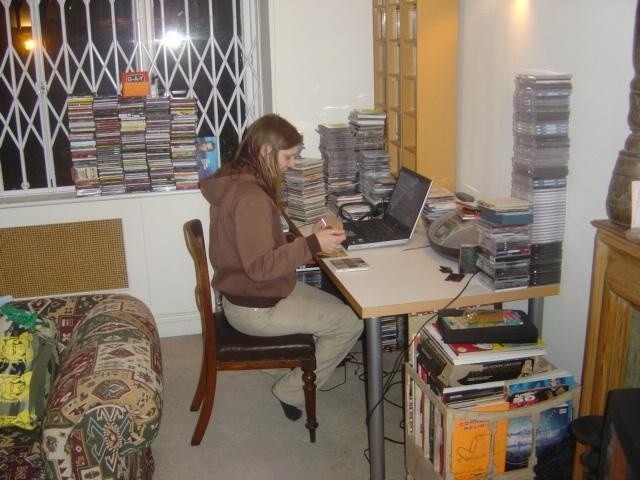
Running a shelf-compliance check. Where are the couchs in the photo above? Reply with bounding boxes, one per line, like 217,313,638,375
0,295,165,480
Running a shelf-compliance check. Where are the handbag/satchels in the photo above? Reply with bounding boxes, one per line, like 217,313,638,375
0,303,60,430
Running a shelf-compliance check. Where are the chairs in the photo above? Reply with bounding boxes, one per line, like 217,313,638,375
183,219,318,446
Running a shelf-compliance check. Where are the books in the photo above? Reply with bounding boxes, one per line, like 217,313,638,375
381,309,575,480
67,94,200,197
295,266,322,289
281,111,397,224
328,258,370,273
421,186,459,223
476,71,572,292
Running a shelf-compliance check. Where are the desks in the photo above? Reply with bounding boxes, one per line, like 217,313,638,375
282,206,560,480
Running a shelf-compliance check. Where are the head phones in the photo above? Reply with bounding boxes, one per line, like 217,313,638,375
339,202,388,222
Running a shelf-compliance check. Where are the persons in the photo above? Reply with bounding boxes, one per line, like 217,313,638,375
197,140,215,154
199,112,364,421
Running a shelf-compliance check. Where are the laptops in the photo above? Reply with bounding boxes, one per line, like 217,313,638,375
339,167,433,252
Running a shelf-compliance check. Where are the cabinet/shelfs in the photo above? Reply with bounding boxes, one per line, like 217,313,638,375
373,1,458,193
